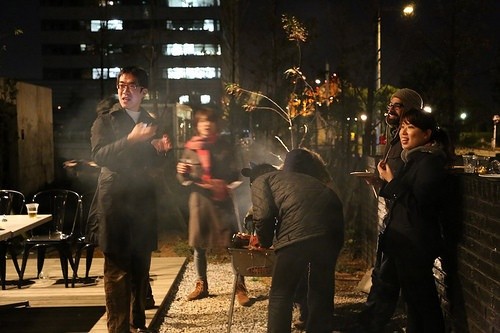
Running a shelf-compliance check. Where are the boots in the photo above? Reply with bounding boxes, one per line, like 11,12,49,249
235,285,250,306
188,278,208,299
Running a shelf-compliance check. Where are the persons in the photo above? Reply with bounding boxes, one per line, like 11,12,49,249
280,149,333,328
354,88,449,333
175,109,250,306
249,163,344,333
82,66,176,333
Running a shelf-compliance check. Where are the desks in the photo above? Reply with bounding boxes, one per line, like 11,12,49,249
0,215,52,286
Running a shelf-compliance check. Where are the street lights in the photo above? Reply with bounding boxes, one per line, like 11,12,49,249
377,2,415,143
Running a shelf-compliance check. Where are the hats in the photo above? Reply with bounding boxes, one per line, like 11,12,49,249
391,88,422,110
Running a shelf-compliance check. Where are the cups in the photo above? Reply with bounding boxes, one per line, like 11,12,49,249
462,154,476,173
26,204,39,219
475,156,490,174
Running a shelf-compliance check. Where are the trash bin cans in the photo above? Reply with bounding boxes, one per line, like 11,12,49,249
61,160,101,236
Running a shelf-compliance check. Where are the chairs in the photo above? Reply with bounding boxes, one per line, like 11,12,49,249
0,189,99,290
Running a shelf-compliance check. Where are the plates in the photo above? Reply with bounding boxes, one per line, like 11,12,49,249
478,173,500,178
350,171,374,176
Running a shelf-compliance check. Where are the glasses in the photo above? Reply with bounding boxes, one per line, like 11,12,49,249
116,84,144,90
386,103,404,110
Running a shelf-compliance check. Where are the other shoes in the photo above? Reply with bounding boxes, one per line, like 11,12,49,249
144,295,156,309
